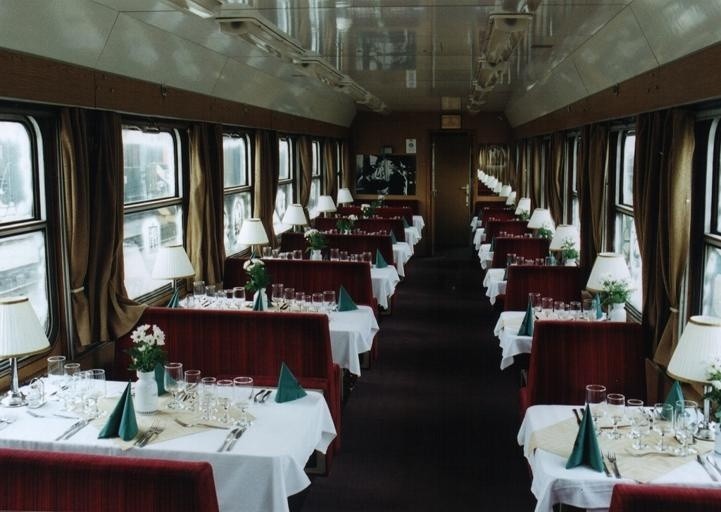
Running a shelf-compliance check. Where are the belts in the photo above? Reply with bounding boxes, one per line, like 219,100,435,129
525,291,598,322
199,376,219,421
25,354,107,427
232,377,254,427
625,398,645,441
605,394,625,440
651,402,674,455
670,399,699,456
181,369,201,413
270,282,337,320
188,279,247,313
214,379,235,423
627,410,650,452
162,362,184,409
584,384,605,439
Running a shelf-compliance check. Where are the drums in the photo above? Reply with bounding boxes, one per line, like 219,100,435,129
696,454,718,483
214,428,239,452
225,428,246,452
63,420,93,439
53,420,80,442
571,408,582,428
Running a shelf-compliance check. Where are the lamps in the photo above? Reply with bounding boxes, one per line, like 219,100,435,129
514,197,531,216
337,188,355,208
214,9,391,115
466,14,532,114
334,2,353,34
505,191,517,206
317,195,337,219
477,168,512,197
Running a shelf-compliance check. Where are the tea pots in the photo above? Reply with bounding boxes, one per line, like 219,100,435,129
477,142,518,198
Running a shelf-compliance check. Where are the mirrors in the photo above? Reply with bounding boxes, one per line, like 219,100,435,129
477,142,518,198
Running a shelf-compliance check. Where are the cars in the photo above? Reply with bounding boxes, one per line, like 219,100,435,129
334,214,402,221
496,230,556,268
326,227,388,237
482,207,514,212
260,246,373,267
347,204,411,209
488,216,520,222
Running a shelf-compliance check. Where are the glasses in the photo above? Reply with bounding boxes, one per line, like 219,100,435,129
214,9,391,115
337,188,355,208
514,197,531,216
466,14,532,114
477,168,512,197
334,2,353,34
505,191,517,206
317,195,337,219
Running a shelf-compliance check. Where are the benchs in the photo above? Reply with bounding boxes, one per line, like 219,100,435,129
0,198,420,512
474,200,721,512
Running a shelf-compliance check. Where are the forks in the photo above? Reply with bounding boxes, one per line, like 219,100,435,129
173,419,231,431
258,390,274,403
137,428,162,447
598,450,611,478
707,452,721,474
608,451,621,479
132,426,156,446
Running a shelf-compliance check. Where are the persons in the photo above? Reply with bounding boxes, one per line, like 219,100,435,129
386,160,404,195
